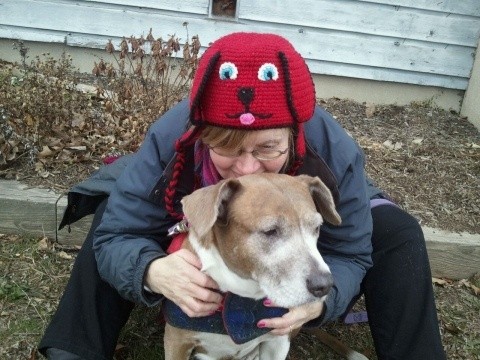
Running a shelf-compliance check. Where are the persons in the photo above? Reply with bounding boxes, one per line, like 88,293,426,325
30,32,451,359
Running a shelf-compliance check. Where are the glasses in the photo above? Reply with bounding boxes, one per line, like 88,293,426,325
207,144,289,161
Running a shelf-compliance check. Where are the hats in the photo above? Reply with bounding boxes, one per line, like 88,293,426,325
164,32,316,221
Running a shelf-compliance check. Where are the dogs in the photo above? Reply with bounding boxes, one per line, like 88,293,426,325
163,173,342,360
